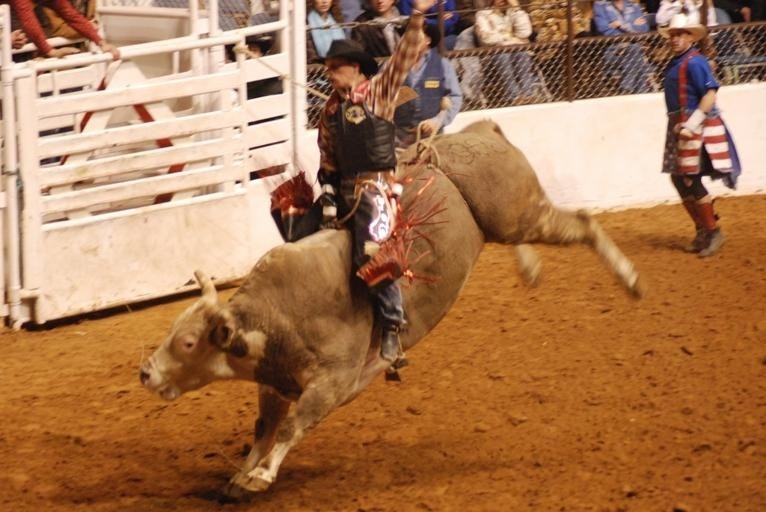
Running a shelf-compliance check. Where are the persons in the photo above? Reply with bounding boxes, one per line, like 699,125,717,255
3,1,120,76
594,1,653,95
225,2,461,124
656,0,765,85
451,0,547,107
395,15,464,147
272,1,435,368
659,11,744,262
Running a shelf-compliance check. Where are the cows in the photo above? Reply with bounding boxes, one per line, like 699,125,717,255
135,115,644,504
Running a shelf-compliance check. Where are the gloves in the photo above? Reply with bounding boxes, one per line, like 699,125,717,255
317,214,340,230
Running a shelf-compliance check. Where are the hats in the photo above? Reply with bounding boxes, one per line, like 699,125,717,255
395,20,439,48
658,15,705,41
307,39,378,75
226,35,273,61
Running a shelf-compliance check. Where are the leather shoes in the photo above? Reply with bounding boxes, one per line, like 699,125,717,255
380,323,399,362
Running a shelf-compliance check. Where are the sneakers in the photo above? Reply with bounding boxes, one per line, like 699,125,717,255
686,229,723,258
